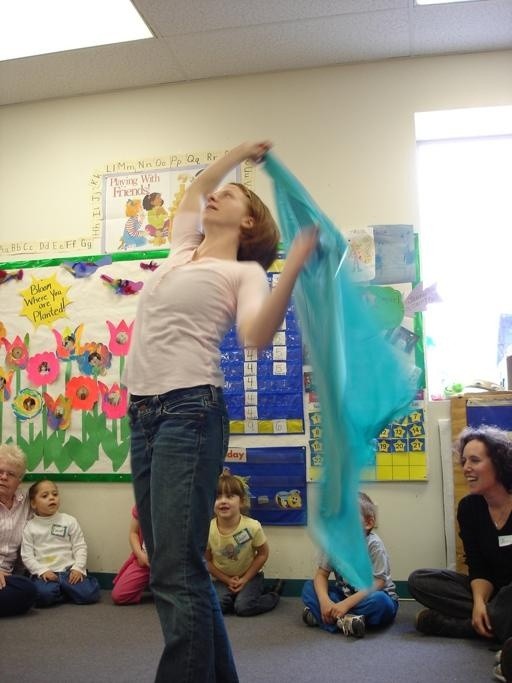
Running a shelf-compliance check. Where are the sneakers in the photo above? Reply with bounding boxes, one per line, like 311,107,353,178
302,607,317,626
335,612,365,637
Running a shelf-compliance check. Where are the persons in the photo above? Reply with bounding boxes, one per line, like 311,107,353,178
204,473,287,617
1,444,39,616
300,488,400,637
19,476,102,606
108,501,150,605
119,136,323,682
406,430,512,644
491,636,511,683
0,329,131,425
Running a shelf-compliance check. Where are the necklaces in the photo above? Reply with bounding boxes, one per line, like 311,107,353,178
486,502,509,528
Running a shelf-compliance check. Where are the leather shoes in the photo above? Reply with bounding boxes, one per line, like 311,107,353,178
416,609,456,639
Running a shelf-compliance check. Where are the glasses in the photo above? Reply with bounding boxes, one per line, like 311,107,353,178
0,469,19,478
